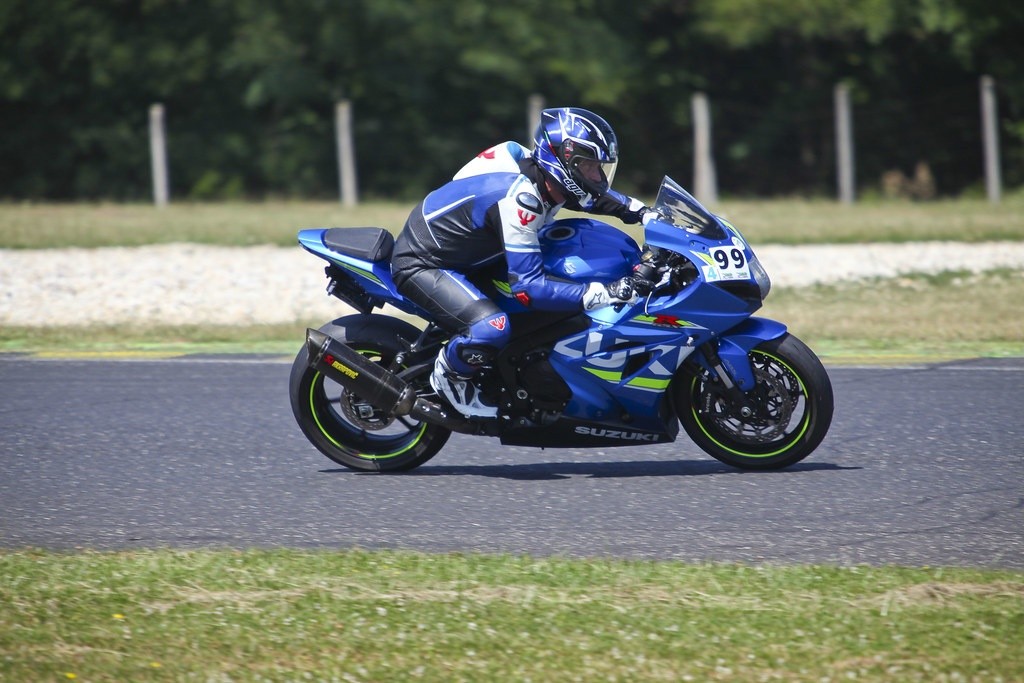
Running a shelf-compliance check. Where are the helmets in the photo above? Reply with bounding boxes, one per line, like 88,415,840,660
530,107,620,211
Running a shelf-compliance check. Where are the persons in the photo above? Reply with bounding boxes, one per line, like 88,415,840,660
392,107,672,423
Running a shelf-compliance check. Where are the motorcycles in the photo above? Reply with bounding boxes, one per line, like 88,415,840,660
288,175,834,474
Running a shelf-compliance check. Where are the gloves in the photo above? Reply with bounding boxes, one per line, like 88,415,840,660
582,277,656,312
622,196,674,226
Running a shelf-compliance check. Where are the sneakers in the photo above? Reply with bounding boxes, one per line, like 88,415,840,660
429,347,498,417
